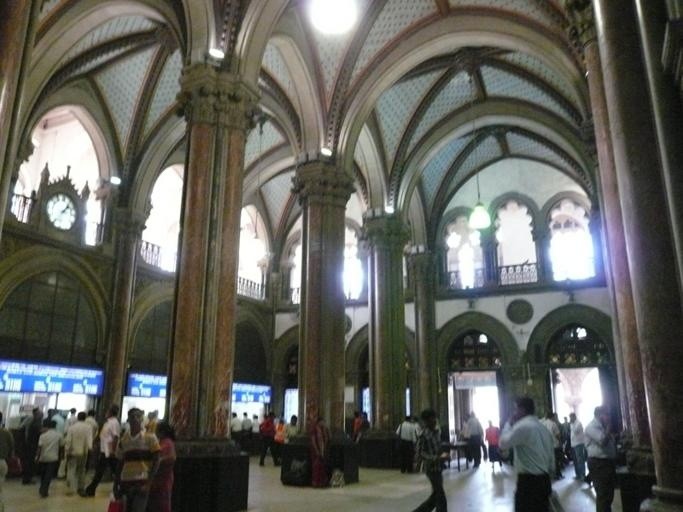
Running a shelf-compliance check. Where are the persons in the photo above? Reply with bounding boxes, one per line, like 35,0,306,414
412,407,451,512
0,410,586,509
497,398,556,512
583,405,619,512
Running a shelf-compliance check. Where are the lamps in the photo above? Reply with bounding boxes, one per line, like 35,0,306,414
465,64,492,231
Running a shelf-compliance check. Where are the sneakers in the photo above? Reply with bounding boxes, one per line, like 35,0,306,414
23,477,95,497
